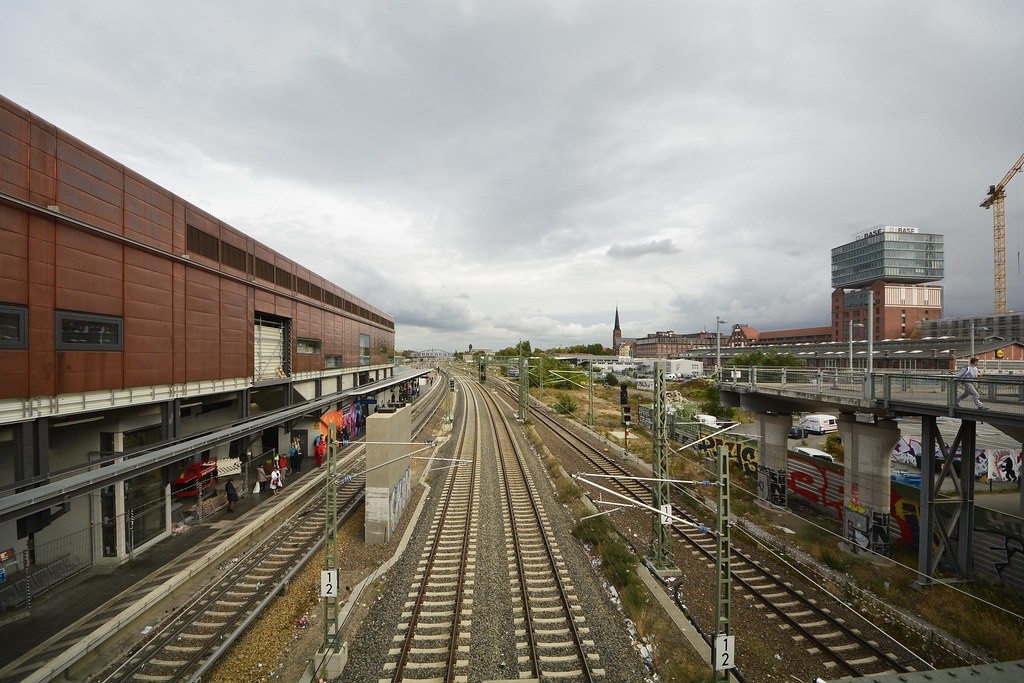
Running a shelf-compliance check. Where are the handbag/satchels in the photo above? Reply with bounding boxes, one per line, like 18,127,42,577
273,480,277,485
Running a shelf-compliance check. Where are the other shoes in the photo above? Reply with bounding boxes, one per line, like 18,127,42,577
274,493,276,495
957,399,962,408
262,491,265,492
276,492,280,493
296,471,301,475
227,508,234,512
978,407,989,410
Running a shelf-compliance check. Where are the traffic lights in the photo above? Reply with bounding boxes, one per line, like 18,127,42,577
620,385,628,405
625,416,630,421
624,407,630,413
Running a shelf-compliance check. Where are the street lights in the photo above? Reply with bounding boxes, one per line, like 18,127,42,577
970,323,989,359
849,319,864,384
716,316,728,381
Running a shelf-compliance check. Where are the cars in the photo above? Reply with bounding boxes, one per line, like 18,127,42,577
788,426,808,439
665,372,676,380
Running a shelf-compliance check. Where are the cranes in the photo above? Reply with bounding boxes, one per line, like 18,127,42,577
980,154,1024,314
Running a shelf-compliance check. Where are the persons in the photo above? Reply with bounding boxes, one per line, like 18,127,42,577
955,358,989,409
256,464,266,492
288,445,304,475
314,434,333,467
402,391,407,400
336,427,350,448
225,479,237,513
270,467,282,495
356,413,366,435
279,454,288,482
411,382,419,396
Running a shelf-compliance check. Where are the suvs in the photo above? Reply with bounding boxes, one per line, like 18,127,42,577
892,470,921,487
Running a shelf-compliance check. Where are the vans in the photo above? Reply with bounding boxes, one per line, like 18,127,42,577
806,415,838,435
793,447,834,463
